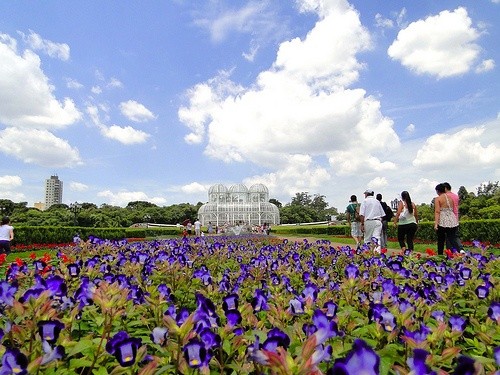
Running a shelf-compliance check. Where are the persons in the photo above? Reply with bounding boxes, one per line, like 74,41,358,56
360,188,381,251
379,196,393,251
441,184,464,255
397,191,417,255
434,184,460,255
0,214,15,258
347,196,364,248
178,220,272,237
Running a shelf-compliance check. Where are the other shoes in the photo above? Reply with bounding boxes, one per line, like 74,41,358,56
356,242,361,250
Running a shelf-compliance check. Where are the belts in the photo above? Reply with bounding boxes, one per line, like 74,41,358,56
366,218,380,220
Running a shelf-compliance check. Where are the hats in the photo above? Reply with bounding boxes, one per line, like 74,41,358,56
363,189,374,194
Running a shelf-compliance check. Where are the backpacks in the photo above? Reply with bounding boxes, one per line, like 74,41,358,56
355,202,361,222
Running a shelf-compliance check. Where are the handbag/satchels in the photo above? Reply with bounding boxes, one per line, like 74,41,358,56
383,202,393,221
398,199,407,220
